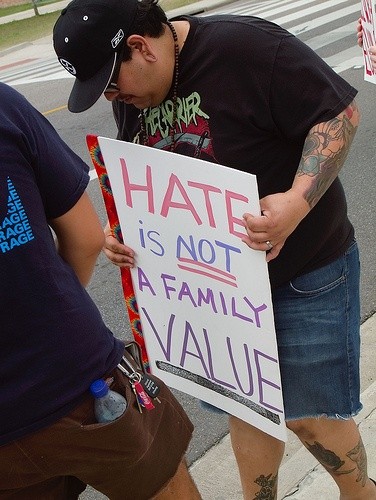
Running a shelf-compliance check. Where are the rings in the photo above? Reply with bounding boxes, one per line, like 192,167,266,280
266,240,274,250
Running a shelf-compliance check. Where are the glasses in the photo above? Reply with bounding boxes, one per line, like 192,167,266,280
105,33,128,93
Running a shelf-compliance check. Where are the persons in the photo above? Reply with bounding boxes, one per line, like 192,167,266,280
0,82,203,500
53,0,375,499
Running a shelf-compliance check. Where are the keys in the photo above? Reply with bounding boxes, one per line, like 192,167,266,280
116,354,163,413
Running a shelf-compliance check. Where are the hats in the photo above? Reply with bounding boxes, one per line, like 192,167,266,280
53,0,139,113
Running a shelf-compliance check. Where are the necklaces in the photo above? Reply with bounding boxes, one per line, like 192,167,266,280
138,20,180,151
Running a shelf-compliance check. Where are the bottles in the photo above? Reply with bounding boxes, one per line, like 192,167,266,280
90,380,127,423
